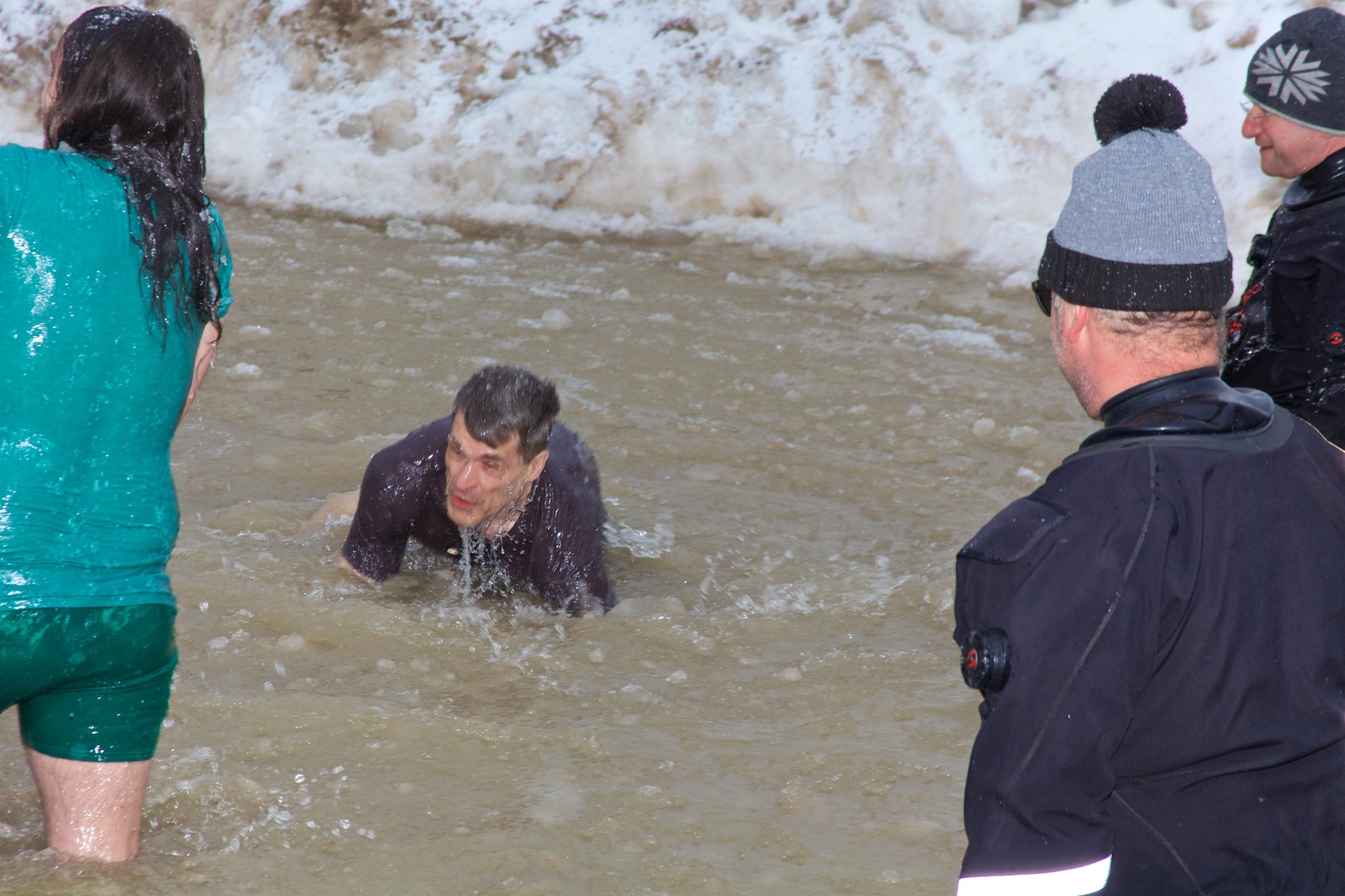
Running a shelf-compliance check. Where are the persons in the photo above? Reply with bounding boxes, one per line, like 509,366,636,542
2,6,232,865
951,75,1345,895
1220,7,1345,452
328,365,617,616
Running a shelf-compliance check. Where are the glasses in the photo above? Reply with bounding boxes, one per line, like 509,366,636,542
1033,280,1051,317
1242,99,1273,121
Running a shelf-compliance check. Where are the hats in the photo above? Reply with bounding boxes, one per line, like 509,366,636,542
1039,73,1234,311
1243,6,1345,134
